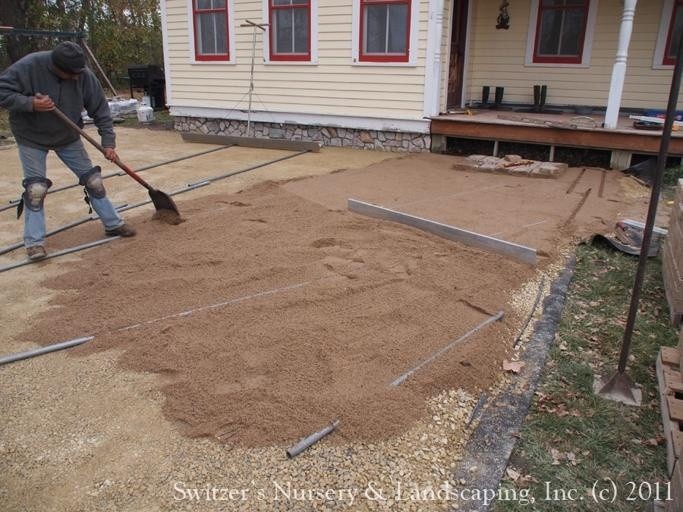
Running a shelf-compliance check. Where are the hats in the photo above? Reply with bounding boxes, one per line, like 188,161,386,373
51,41,86,75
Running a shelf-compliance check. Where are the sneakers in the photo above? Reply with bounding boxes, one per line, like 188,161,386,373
105,223,135,236
27,245,46,259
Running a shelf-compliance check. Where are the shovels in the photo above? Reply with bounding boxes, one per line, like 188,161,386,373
36,93,180,217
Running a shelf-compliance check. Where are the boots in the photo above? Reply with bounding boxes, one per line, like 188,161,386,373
480,86,490,108
490,87,503,106
539,85,546,112
528,86,540,112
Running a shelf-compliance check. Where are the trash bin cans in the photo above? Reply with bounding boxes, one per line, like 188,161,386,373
128,65,163,112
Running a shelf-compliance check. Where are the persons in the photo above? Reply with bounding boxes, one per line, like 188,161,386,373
0,41,136,259
